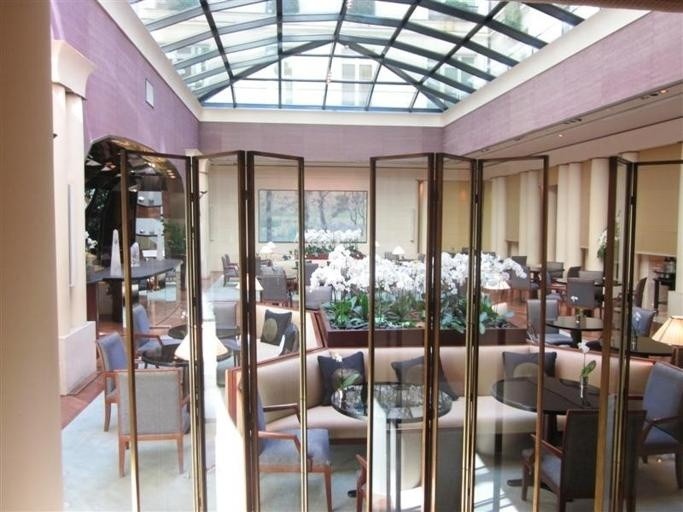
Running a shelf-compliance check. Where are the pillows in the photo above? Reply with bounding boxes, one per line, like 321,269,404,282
393,350,456,400
501,348,557,382
626,308,658,332
261,308,291,347
319,351,368,407
275,324,299,356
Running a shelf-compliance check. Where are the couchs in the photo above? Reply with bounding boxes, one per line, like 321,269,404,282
232,303,325,372
235,345,683,441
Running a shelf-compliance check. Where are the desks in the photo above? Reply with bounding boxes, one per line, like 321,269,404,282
331,383,452,497
143,344,230,406
490,379,611,487
599,334,675,361
168,323,238,368
550,315,607,346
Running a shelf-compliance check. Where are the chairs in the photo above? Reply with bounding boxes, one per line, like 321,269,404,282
236,378,332,512
524,299,570,345
89,248,683,307
96,331,128,433
630,361,681,492
521,410,646,512
131,304,174,368
354,427,421,512
113,368,198,478
